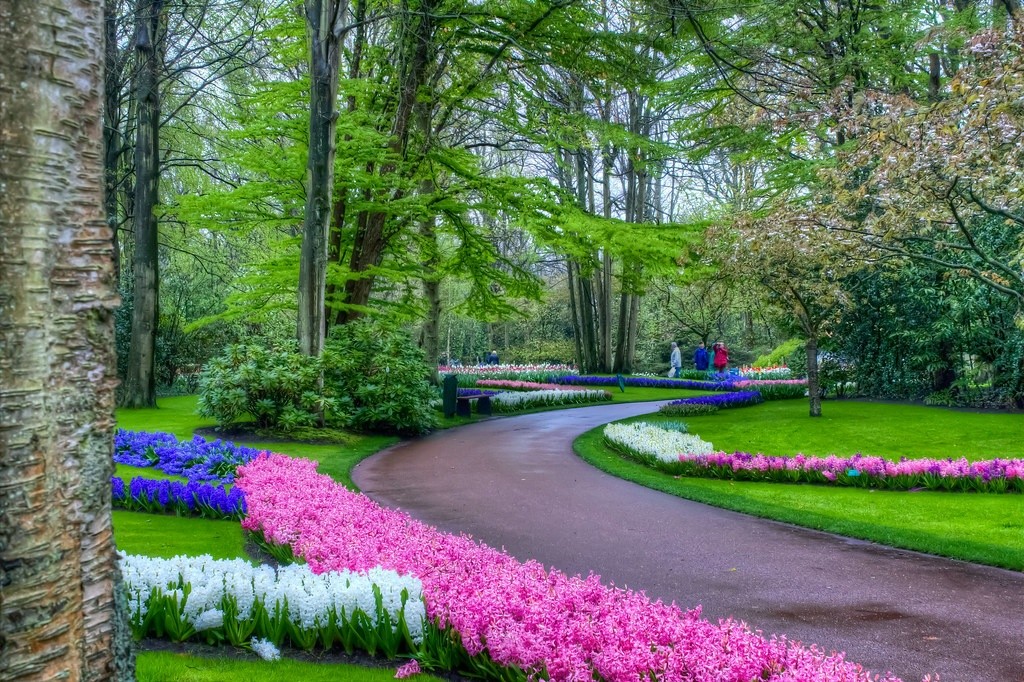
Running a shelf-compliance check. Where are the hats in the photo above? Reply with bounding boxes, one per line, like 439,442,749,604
699,341,703,344
671,343,677,348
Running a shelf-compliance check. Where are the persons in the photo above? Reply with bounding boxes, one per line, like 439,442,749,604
670,342,681,378
695,342,729,373
488,351,499,365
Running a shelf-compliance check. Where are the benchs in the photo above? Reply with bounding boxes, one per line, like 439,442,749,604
456,395,493,418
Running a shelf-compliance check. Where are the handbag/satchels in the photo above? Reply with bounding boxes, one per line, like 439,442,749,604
667,367,676,378
